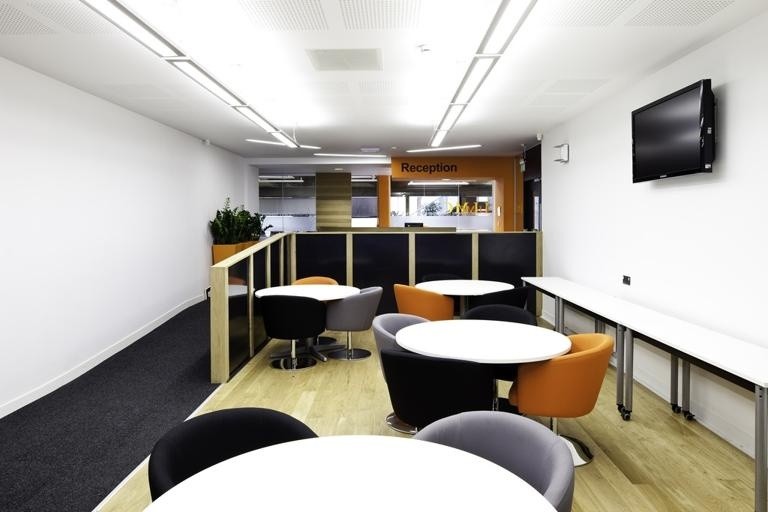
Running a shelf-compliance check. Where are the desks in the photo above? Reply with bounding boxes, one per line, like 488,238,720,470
520,277,767,511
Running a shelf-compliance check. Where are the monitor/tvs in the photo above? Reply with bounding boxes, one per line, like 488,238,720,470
631,79,716,184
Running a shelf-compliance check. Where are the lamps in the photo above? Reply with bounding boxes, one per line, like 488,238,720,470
313,153,387,157
245,139,322,151
79,0,299,150
427,0,538,148
406,144,482,153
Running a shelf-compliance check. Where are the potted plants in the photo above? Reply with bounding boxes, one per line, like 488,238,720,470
209,195,274,265
